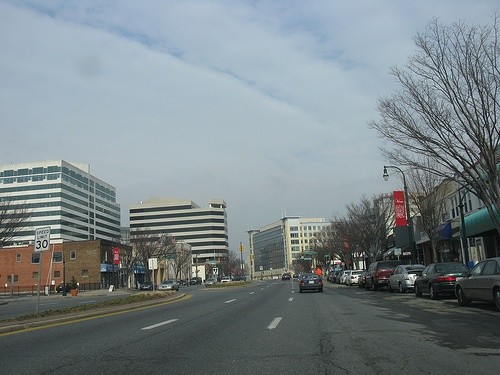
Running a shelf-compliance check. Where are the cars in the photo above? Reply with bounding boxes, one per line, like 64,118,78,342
298,272,323,293
158,278,181,292
281,273,292,281
56,282,78,294
455,257,500,312
386,264,427,294
204,277,216,287
414,262,470,300
293,273,299,278
140,281,155,291
220,276,232,284
326,268,366,288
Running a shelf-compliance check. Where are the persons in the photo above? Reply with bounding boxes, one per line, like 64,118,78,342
316,268,322,278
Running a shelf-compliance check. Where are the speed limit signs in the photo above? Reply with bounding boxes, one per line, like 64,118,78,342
34,227,50,252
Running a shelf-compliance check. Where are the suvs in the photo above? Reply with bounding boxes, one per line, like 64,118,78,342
362,259,402,292
190,277,203,287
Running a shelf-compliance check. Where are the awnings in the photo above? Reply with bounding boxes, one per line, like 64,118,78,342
431,222,451,243
459,199,500,239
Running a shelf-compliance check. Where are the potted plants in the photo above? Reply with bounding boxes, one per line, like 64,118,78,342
68,276,79,296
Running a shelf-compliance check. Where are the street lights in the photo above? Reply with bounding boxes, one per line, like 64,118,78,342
382,164,426,265
